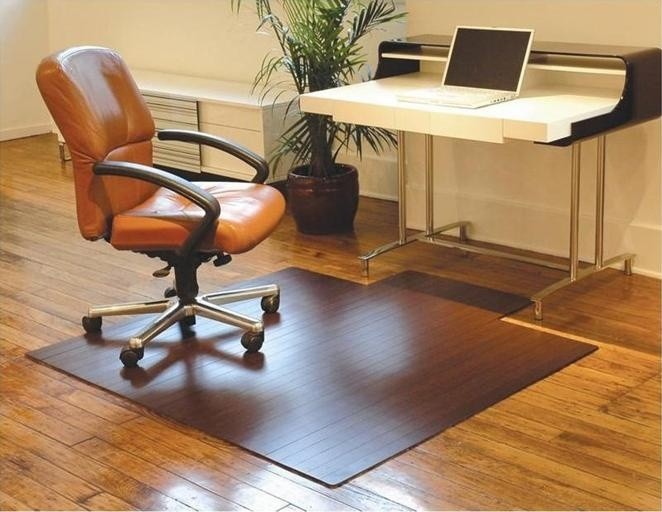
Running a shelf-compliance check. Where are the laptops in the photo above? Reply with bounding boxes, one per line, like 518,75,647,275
395,25,535,109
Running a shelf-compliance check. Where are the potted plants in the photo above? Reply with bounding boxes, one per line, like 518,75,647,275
229,0,410,234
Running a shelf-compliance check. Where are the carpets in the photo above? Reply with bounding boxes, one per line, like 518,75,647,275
25,265,601,486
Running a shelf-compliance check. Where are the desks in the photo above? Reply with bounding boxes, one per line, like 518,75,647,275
299,32,661,321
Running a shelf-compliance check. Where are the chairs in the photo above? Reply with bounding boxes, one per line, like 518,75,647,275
36,46,288,366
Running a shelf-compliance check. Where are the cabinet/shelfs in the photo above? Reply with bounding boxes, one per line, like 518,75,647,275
58,71,313,186
